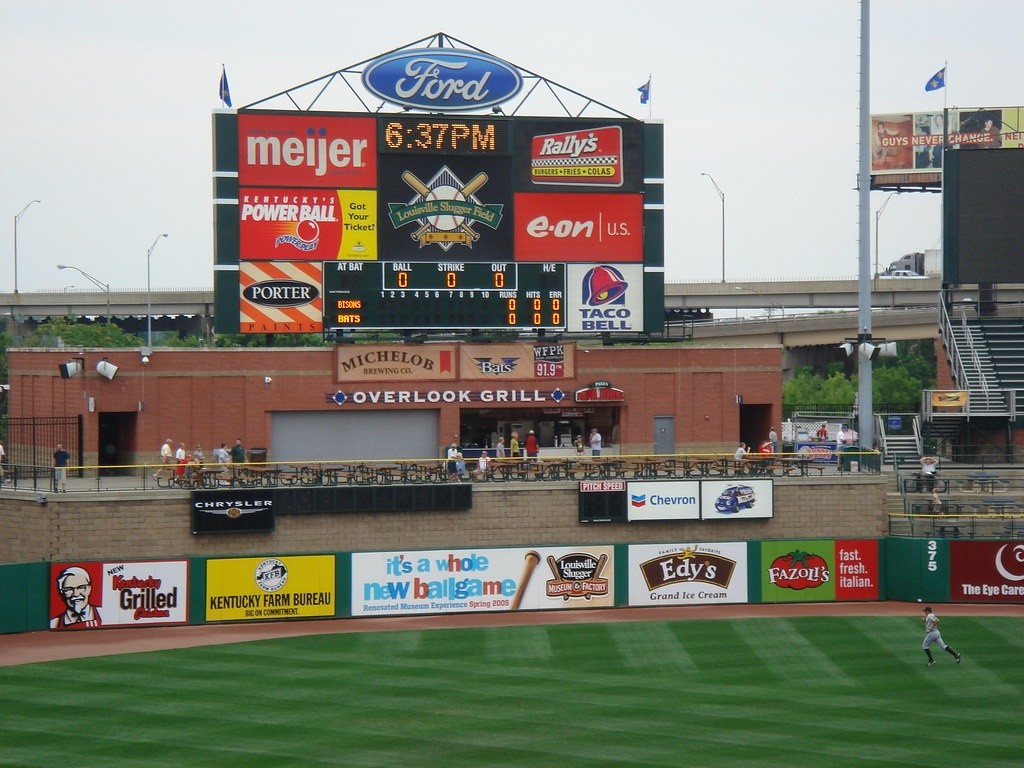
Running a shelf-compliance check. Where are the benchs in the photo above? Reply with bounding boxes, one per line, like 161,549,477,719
156,465,826,489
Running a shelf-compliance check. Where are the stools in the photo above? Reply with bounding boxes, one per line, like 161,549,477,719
904,475,1024,538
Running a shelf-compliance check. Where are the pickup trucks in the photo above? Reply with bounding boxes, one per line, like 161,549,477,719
880,269,929,280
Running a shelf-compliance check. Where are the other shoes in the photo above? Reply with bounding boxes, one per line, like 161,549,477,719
55,488,57,493
152,473,158,481
956,653,961,663
63,490,65,493
927,660,936,667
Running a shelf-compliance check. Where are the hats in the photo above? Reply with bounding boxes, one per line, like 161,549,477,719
529,430,534,435
822,425,825,427
841,426,848,430
922,607,932,613
591,429,597,432
166,439,172,442
576,435,581,437
451,443,457,448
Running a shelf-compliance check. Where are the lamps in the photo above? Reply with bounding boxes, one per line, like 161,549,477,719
492,105,507,119
97,357,121,381
59,357,86,378
398,103,416,115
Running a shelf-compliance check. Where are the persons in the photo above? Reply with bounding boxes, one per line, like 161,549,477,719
590,428,602,468
817,424,829,441
218,443,230,487
510,432,521,458
836,426,848,471
921,607,961,667
526,429,539,470
52,444,70,493
496,437,506,458
769,427,779,457
472,451,492,482
448,443,465,482
0,439,9,485
574,435,585,467
932,488,941,512
734,443,760,474
152,439,174,481
759,441,774,474
176,442,211,489
227,439,248,486
915,456,939,494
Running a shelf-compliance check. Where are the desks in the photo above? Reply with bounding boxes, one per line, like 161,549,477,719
905,477,950,494
1003,525,1023,538
982,498,1014,521
922,498,955,514
931,522,966,537
197,458,813,490
967,474,999,493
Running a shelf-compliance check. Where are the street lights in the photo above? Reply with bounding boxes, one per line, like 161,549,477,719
13,198,43,293
57,264,111,328
147,232,170,348
875,192,901,279
701,172,726,282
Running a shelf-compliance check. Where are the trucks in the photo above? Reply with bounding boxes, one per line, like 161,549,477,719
880,248,943,279
